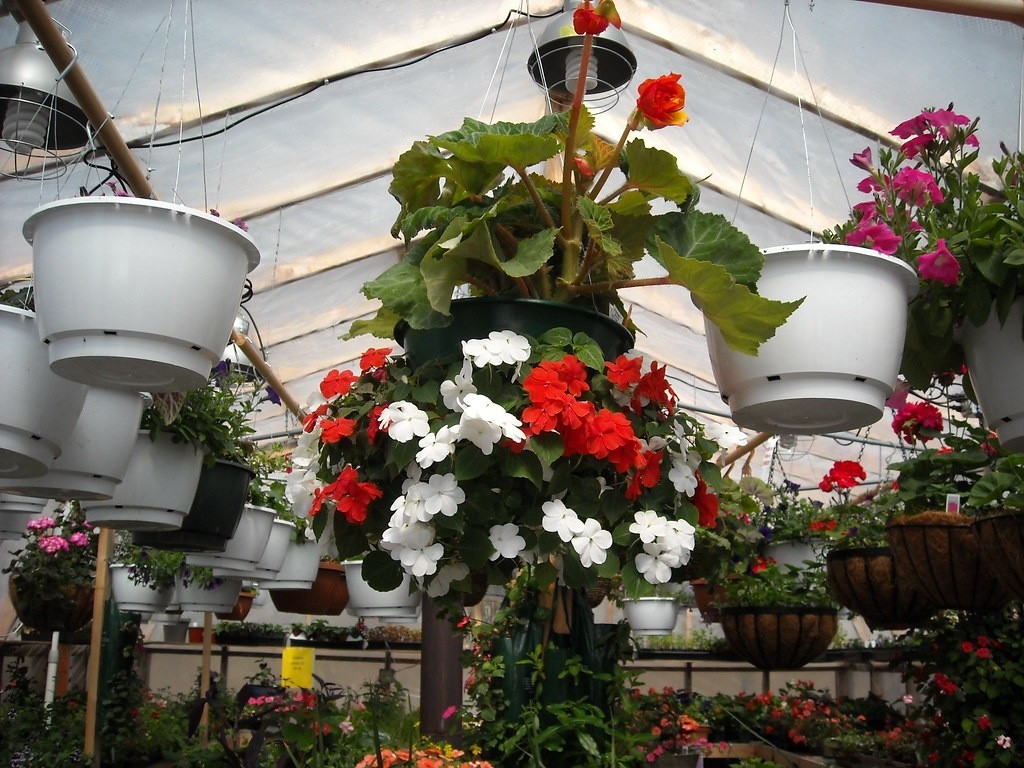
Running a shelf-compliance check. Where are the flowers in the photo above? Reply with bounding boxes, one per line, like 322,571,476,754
2,499,100,618
622,679,898,761
142,359,281,465
285,0,1024,648
107,180,250,232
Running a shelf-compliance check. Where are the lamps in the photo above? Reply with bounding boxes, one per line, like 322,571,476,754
0,17,102,181
209,311,264,387
527,0,638,116
775,432,813,462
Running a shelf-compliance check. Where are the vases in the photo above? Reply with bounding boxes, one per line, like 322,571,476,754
654,753,701,768
718,604,838,673
7,572,95,634
174,569,244,612
706,243,921,434
883,509,1011,615
819,542,940,630
132,456,255,554
484,623,623,756
853,752,911,768
0,303,203,538
20,195,262,395
952,297,1024,453
971,511,1024,610
389,297,636,382
687,723,711,742
585,577,608,606
692,575,750,623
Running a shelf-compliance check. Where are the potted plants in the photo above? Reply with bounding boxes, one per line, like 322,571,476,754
184,474,423,621
213,620,421,646
215,584,260,621
238,657,278,700
109,548,183,614
610,585,695,637
626,633,737,661
827,633,905,662
